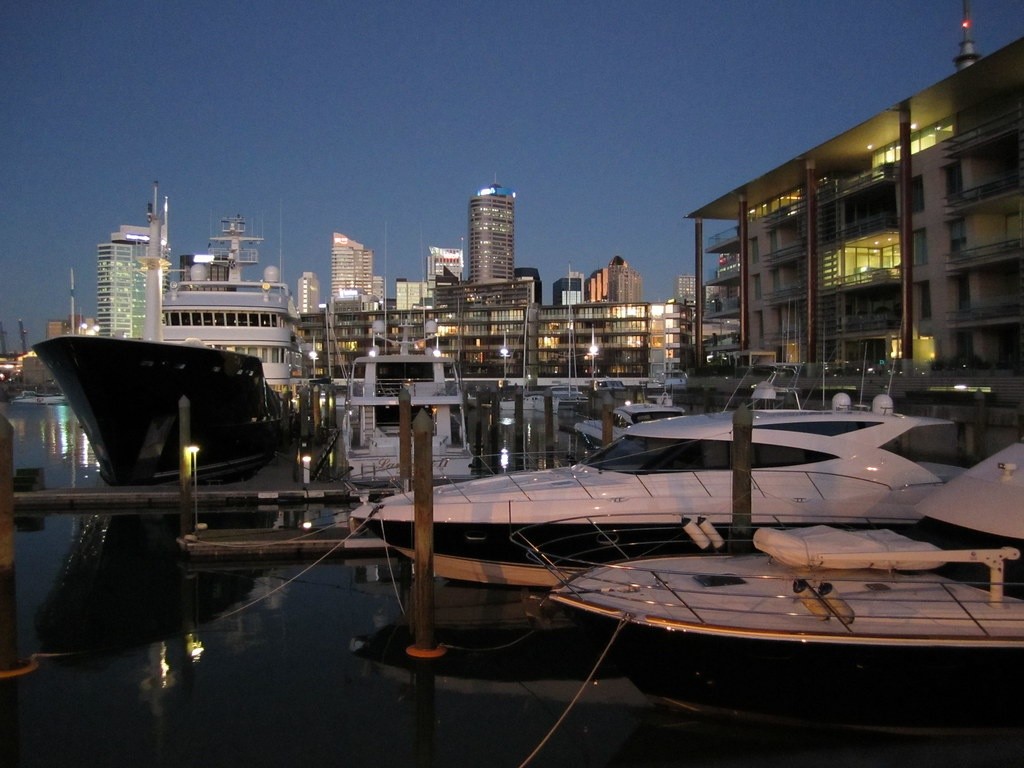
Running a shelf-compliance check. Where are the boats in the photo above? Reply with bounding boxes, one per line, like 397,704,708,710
26,178,309,488
352,576,656,706
340,219,473,488
571,362,687,457
505,440,1024,743
342,327,972,592
31,456,302,670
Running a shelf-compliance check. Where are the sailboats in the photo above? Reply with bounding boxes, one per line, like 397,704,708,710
497,263,588,412
